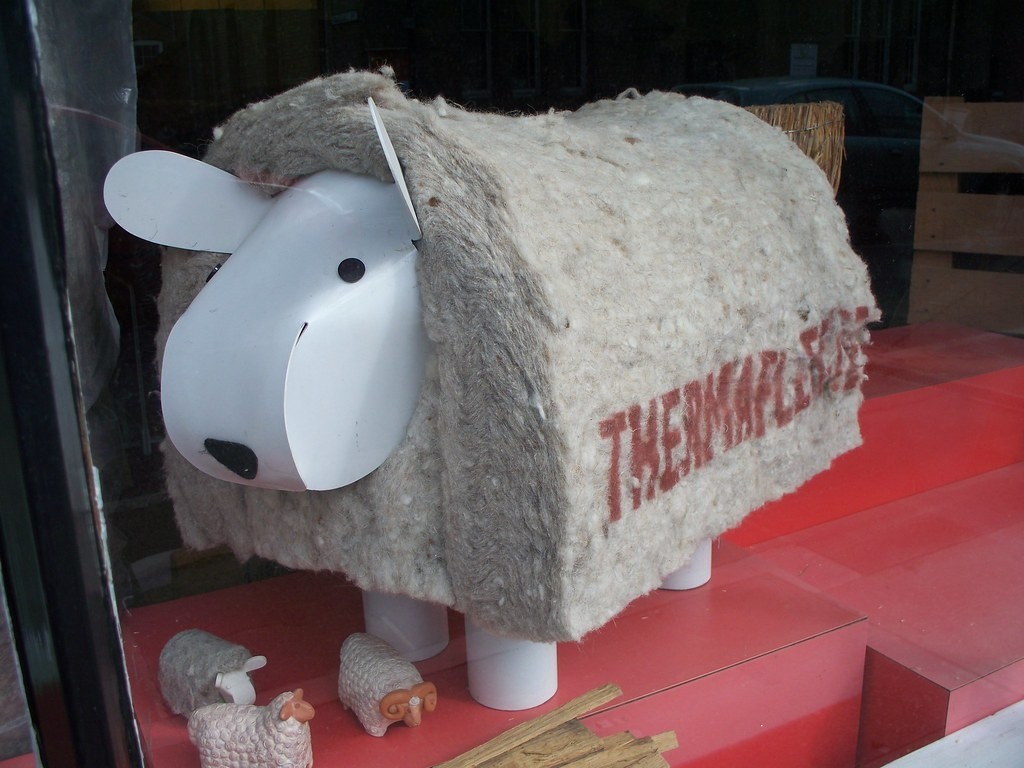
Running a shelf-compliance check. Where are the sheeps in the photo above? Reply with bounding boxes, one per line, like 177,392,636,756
187,687,315,768
337,632,437,737
102,70,882,711
159,629,267,720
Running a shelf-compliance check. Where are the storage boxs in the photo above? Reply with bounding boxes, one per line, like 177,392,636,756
905,96,1024,329
